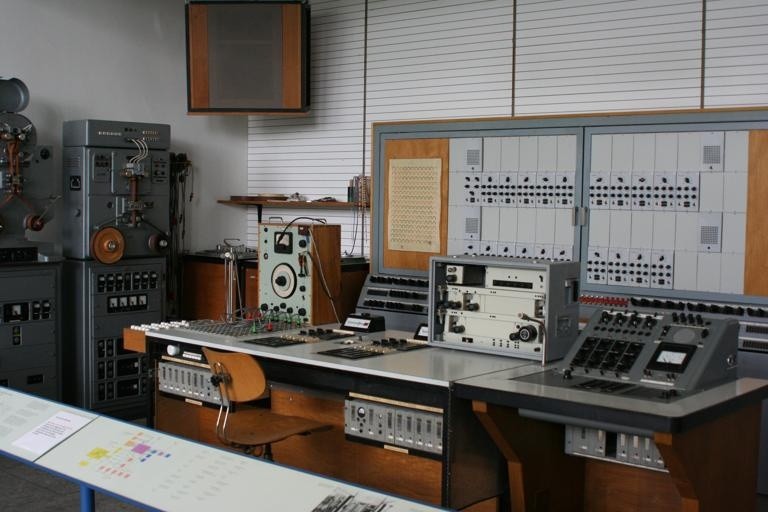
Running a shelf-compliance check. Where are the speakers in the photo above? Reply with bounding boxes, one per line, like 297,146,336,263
184,0,311,114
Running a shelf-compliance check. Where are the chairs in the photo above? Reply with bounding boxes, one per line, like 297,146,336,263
201,347,333,461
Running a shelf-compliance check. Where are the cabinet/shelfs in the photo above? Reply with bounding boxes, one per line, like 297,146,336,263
179,254,369,325
